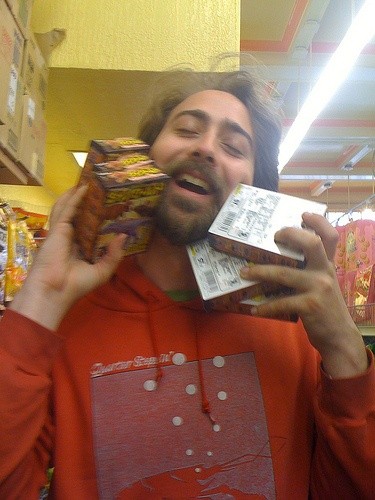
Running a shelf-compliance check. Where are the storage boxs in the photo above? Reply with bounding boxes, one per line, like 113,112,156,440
0,0,50,187
186,183,329,323
74,136,171,264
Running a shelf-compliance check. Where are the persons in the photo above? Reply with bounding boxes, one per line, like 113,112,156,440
0,52,375,500
